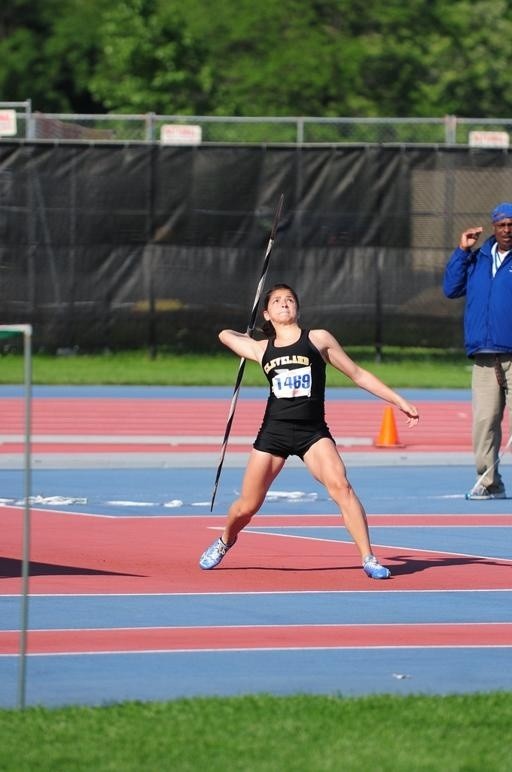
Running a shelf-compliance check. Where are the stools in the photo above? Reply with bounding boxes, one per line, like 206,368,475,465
475,352,507,390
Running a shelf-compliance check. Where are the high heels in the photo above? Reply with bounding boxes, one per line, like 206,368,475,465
373,405,406,447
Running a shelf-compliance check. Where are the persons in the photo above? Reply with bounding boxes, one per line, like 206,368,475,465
441,201,510,500
199,283,420,580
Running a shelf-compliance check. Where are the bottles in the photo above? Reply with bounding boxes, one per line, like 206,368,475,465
491,202,511,222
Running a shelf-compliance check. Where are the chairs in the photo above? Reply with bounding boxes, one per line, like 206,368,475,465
466,484,506,500
363,555,391,578
199,533,237,570
